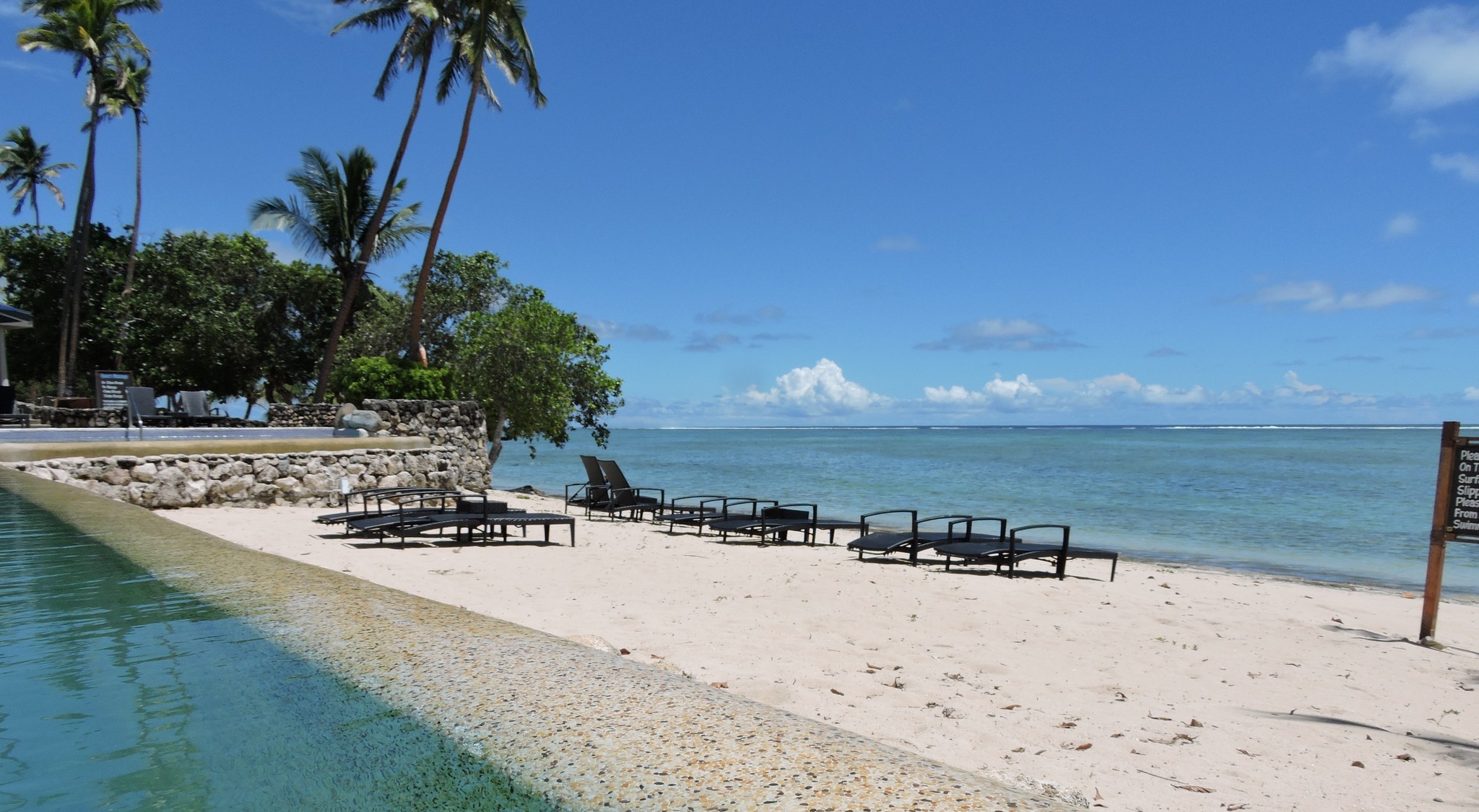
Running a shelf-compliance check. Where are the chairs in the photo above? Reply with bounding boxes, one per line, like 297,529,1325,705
179,390,233,429
125,387,173,430
564,456,1119,582
316,485,575,546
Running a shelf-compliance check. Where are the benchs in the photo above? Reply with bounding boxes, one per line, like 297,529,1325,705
0,414,30,429
